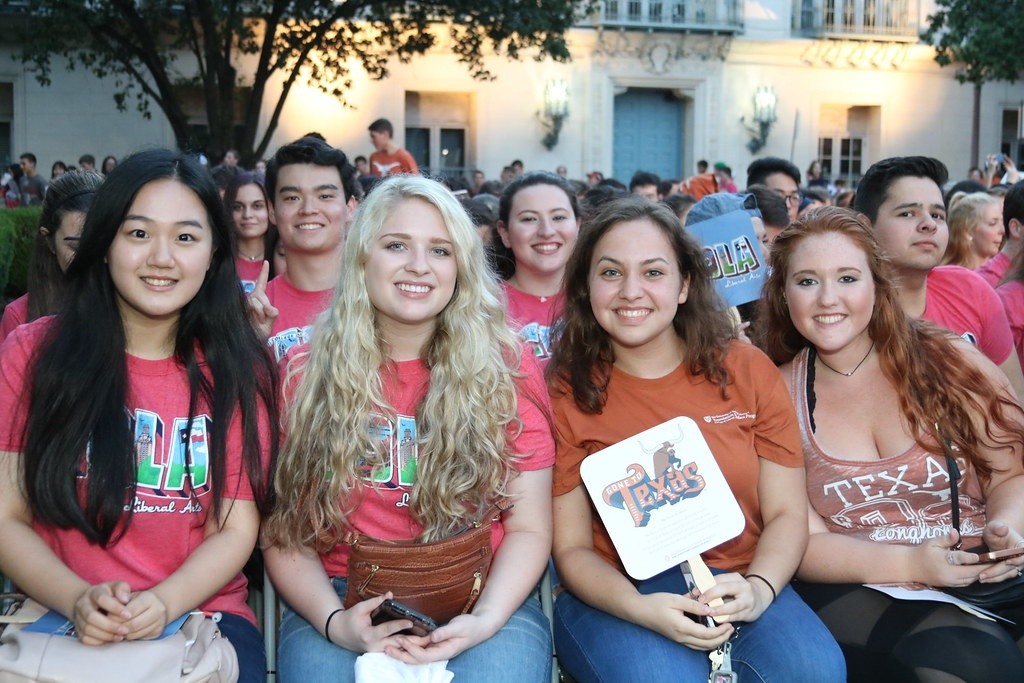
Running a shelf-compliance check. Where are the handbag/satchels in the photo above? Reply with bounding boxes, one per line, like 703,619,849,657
0,599,239,683
345,521,494,626
940,546,1024,606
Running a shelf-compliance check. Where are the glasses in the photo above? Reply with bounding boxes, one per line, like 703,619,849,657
775,192,803,207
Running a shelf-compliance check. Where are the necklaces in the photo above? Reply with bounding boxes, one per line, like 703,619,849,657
240,250,263,261
817,341,874,376
537,297,548,302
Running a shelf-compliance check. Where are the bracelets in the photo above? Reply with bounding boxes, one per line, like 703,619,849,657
745,574,775,601
325,609,345,643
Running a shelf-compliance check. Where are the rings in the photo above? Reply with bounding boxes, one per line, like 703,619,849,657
1017,568,1022,576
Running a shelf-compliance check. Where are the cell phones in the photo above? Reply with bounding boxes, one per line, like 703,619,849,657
369,598,437,637
978,546,1024,564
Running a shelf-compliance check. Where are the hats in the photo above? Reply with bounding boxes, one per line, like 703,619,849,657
684,193,762,228
587,172,603,181
470,193,500,221
447,177,469,195
945,177,991,216
599,179,625,192
714,162,725,169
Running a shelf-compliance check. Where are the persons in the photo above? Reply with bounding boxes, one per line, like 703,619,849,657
546,198,847,683
368,119,418,176
627,158,854,346
442,160,627,281
0,148,273,683
247,137,359,449
854,156,1024,408
0,170,104,343
257,177,556,683
940,154,1024,369
0,153,116,208
754,206,1024,683
355,156,369,176
209,148,287,294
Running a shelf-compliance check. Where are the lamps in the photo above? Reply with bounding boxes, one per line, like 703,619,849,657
538,76,570,150
739,88,779,154
